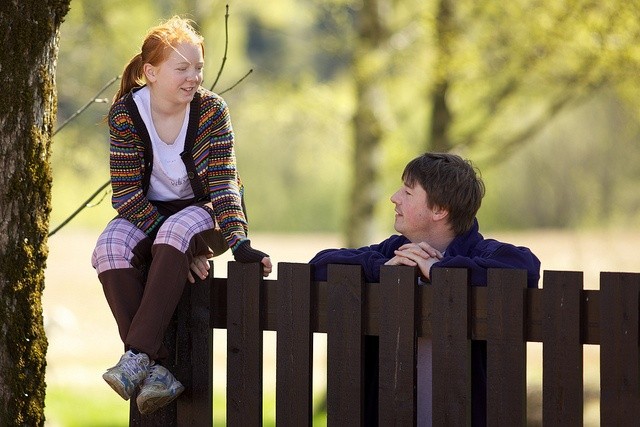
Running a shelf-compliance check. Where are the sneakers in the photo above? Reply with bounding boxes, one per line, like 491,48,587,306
102,349,155,401
136,360,185,416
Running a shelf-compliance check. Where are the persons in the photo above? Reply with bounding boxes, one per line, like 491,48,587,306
309,152,541,427
92,15,272,415
